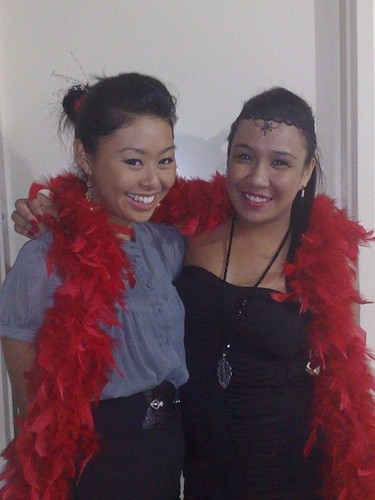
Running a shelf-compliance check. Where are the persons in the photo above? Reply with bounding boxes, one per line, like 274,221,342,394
11,86,326,499
1,72,189,500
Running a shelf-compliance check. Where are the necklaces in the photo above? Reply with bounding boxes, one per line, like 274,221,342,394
214,214,294,390
